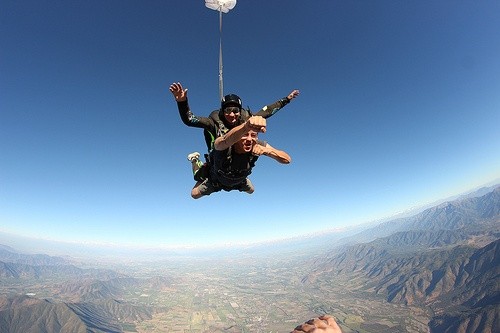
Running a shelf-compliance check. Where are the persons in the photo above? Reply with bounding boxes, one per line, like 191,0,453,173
169,81,300,183
290,314,342,332
189,114,291,199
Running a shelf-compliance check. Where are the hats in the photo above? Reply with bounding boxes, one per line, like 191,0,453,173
221,93,242,106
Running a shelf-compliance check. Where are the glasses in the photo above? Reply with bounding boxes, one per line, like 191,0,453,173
224,108,241,113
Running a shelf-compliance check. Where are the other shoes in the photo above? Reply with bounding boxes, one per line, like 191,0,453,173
186,152,201,160
